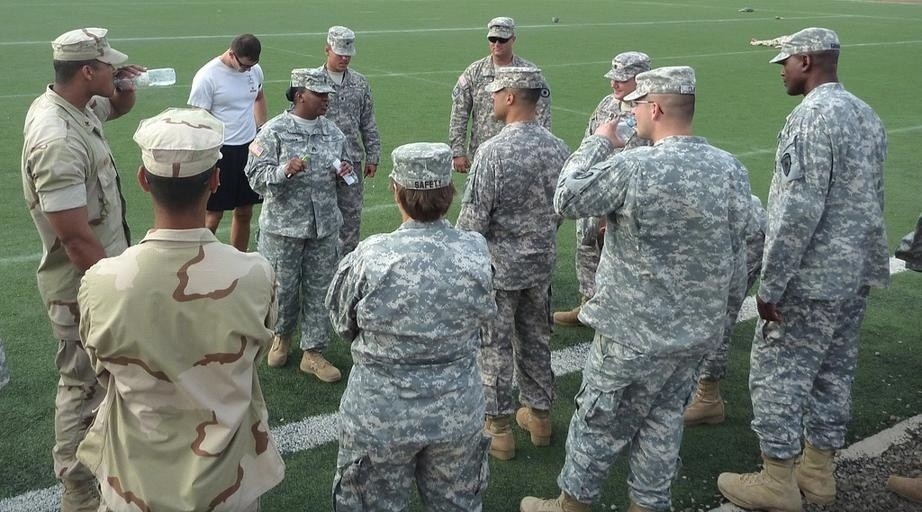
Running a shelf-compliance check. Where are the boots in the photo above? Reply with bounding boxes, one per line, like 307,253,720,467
482,415,515,460
717,459,802,512
300,350,341,382
60,477,100,512
516,407,552,446
268,335,289,368
796,441,836,505
683,381,725,425
629,504,652,512
887,475,921,504
519,492,590,512
553,295,590,325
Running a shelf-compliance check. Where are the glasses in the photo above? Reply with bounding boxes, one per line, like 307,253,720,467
631,101,664,115
489,36,513,43
234,53,259,69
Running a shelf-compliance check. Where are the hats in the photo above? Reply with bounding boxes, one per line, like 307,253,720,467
328,26,355,56
487,16,514,39
388,141,454,189
52,28,128,65
769,28,840,63
133,107,225,178
603,51,651,82
290,68,337,94
485,67,542,93
623,66,696,101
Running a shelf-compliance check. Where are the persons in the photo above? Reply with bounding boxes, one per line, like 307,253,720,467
449,13,552,174
518,66,750,512
885,207,922,501
579,195,772,426
549,51,653,326
186,33,266,254
326,142,497,512
314,25,380,257
75,107,285,512
456,66,572,465
21,28,147,512
717,28,887,511
243,67,359,383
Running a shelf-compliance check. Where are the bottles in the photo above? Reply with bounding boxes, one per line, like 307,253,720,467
114,68,177,87
326,153,358,186
614,116,638,145
